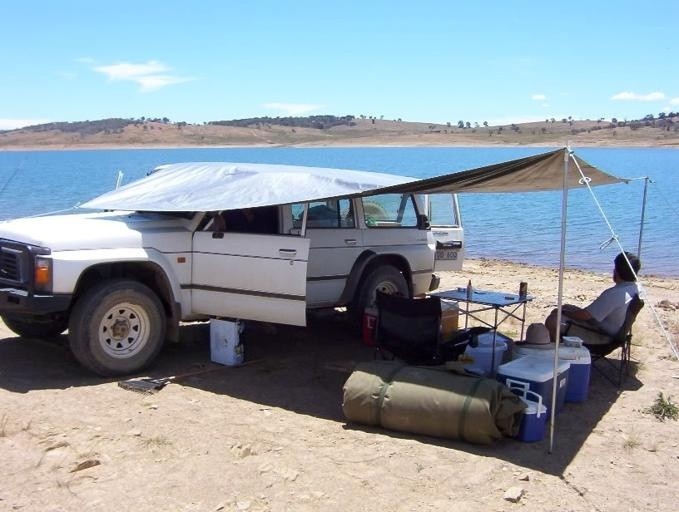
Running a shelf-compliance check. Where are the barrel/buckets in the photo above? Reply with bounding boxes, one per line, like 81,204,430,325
362,307,381,348
440,299,460,344
464,333,509,375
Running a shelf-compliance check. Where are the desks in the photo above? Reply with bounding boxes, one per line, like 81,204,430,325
425,286,537,375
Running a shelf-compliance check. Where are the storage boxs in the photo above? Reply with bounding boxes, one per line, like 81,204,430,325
460,329,592,443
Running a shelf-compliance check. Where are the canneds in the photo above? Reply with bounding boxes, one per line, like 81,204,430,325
520,282,527,297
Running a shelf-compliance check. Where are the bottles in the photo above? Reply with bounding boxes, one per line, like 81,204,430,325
466,280,473,299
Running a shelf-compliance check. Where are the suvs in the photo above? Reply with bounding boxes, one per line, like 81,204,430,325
1,179,466,380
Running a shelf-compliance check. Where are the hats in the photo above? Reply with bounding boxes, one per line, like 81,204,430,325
512,323,555,350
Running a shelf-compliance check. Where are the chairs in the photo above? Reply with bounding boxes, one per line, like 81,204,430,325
375,288,479,366
561,292,643,388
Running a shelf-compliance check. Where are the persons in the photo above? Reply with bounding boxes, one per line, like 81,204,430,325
545,252,641,343
209,207,277,233
